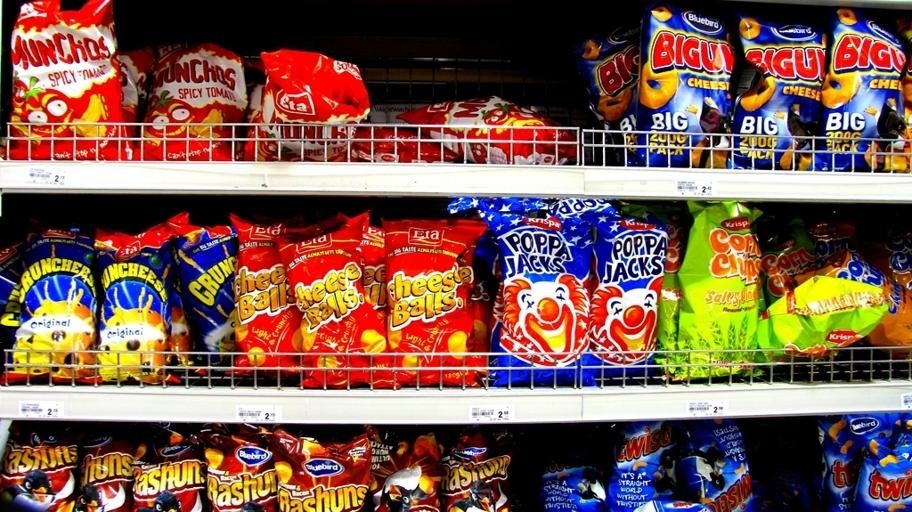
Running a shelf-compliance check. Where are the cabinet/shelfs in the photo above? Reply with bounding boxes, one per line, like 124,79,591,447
2,122,912,204
2,349,912,426
